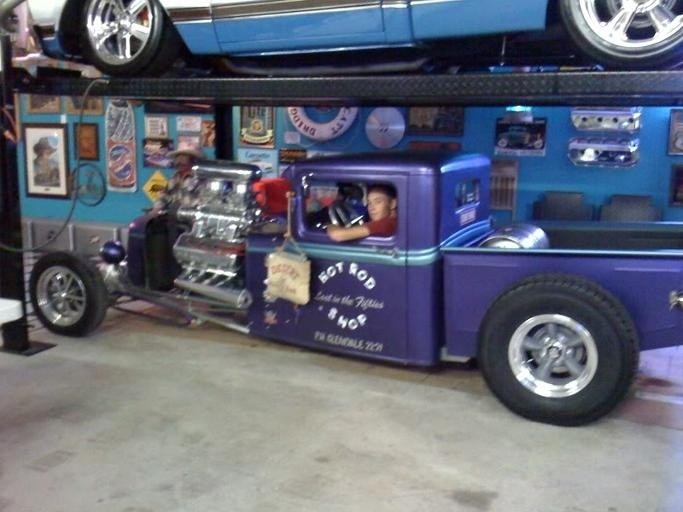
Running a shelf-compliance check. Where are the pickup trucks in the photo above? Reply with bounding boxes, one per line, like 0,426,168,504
28,151,683,427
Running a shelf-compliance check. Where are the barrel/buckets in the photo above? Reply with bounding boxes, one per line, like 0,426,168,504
478,223,550,249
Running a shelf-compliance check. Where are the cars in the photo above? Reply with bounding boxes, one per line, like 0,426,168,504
55,0,683,74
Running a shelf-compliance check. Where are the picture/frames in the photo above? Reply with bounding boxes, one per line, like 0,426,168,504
23,94,104,199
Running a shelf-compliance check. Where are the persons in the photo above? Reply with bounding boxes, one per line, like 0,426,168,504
166,139,205,184
326,185,397,242
34,138,59,188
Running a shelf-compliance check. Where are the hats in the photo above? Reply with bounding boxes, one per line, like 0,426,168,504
164,141,204,158
33,137,57,154
